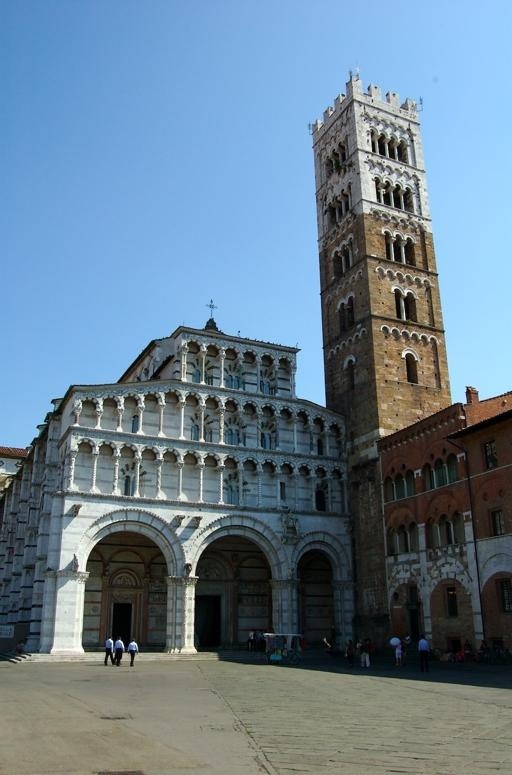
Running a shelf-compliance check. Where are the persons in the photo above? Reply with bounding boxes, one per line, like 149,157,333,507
417,635,432,673
432,634,511,668
247,626,419,672
103,634,116,666
113,636,126,666
127,638,139,666
